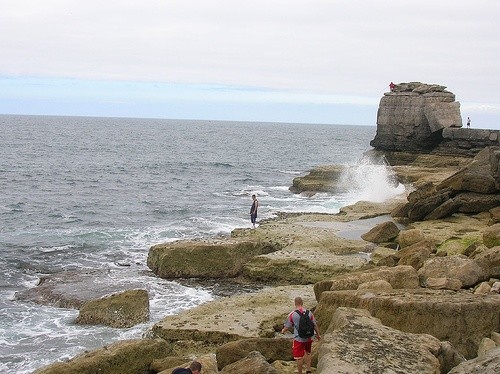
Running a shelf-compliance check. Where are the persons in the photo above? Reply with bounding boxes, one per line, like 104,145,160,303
467,116,471,129
250,194,258,230
389,82,395,92
281,296,322,374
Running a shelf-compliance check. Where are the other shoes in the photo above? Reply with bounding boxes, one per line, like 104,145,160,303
251,226,255,229
306,370,311,374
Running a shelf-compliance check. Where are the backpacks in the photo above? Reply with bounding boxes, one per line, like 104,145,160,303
292,309,314,338
